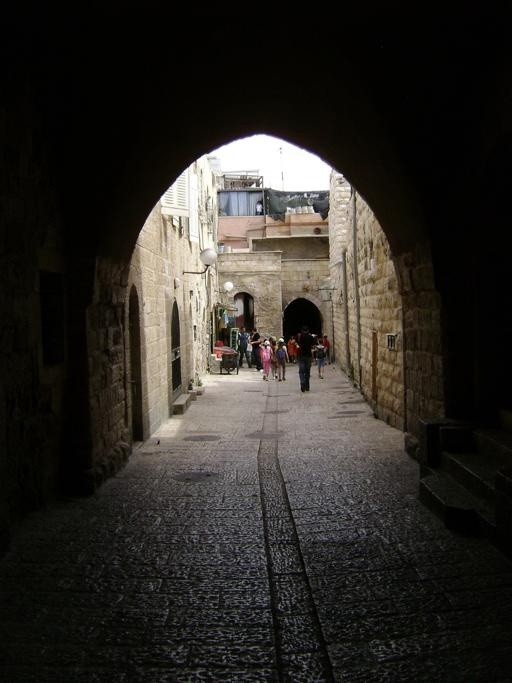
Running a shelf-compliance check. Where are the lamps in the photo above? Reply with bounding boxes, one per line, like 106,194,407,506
214,281,234,294
183,248,217,274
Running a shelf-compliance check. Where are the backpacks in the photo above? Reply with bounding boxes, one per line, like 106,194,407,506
315,346,326,359
276,348,285,361
261,346,270,362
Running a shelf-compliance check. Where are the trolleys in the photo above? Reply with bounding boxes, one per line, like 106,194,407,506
219,351,239,375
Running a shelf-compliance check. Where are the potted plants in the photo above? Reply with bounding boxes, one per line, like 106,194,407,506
287,199,314,214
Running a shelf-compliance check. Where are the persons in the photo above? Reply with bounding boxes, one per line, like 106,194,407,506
237,326,330,392
255,201,263,215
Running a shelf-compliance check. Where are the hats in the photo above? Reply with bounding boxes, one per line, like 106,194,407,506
265,336,284,345
